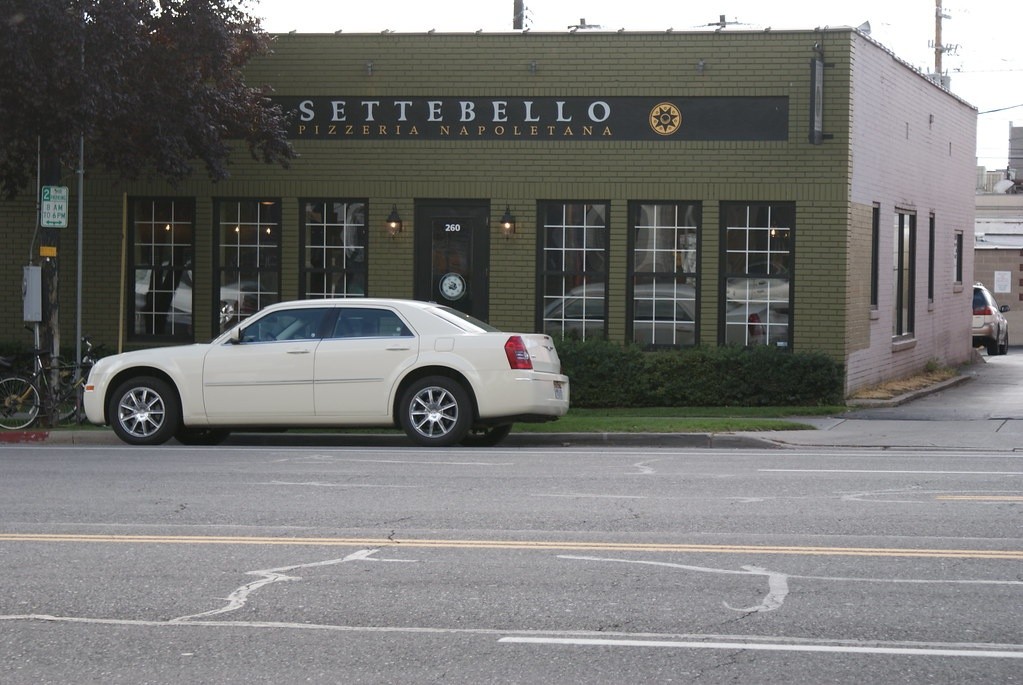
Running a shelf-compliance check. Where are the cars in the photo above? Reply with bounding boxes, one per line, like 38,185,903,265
134,262,269,330
84,296,571,447
545,279,790,350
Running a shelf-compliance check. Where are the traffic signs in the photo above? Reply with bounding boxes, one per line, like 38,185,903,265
41,186,68,229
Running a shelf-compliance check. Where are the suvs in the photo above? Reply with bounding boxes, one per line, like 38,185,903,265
970,282,1009,356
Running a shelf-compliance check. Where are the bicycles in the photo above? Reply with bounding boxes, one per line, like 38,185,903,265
0,325,107,430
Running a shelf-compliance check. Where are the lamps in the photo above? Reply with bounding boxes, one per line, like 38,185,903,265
500,204,516,242
385,203,403,241
531,62,537,73
699,61,703,72
367,63,372,76
